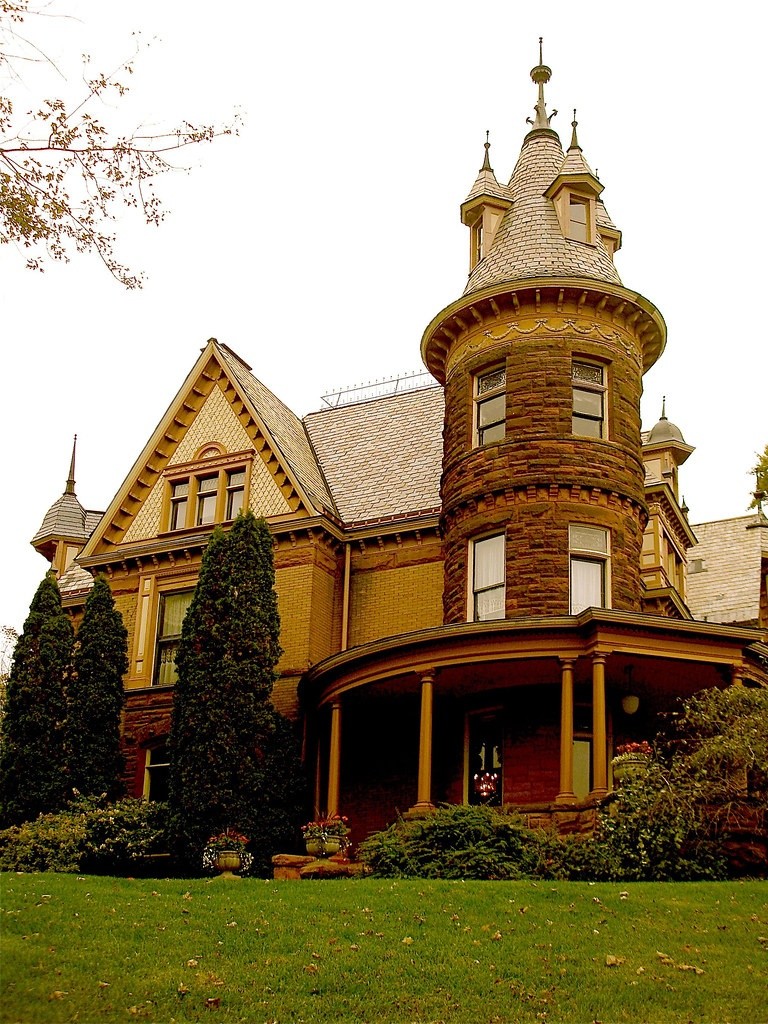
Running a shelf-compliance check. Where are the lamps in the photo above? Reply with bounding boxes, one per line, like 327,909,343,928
623,664,640,714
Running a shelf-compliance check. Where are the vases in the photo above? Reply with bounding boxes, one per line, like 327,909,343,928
613,760,647,779
219,851,240,869
211,855,219,869
305,836,321,852
322,835,340,852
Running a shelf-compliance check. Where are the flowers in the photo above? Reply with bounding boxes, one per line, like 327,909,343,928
300,807,353,852
610,741,652,764
202,830,254,872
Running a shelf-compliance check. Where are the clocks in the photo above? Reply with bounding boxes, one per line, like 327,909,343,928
200,449,220,459
